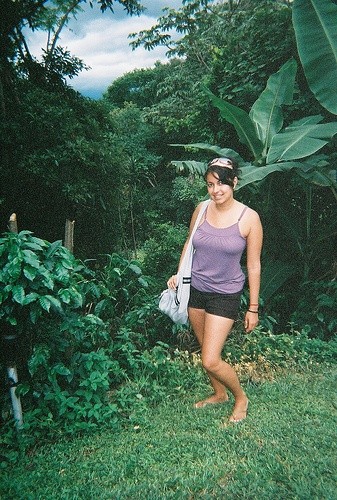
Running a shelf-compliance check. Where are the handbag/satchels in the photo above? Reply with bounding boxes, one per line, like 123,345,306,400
157,240,194,325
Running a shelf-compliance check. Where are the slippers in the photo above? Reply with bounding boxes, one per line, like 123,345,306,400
195,394,229,409
229,398,249,423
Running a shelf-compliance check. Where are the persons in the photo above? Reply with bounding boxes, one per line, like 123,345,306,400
167,156,260,423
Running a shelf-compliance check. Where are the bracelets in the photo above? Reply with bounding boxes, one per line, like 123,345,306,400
249,304,260,307
248,310,259,313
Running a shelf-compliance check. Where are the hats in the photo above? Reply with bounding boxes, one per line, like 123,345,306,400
207,156,234,170
